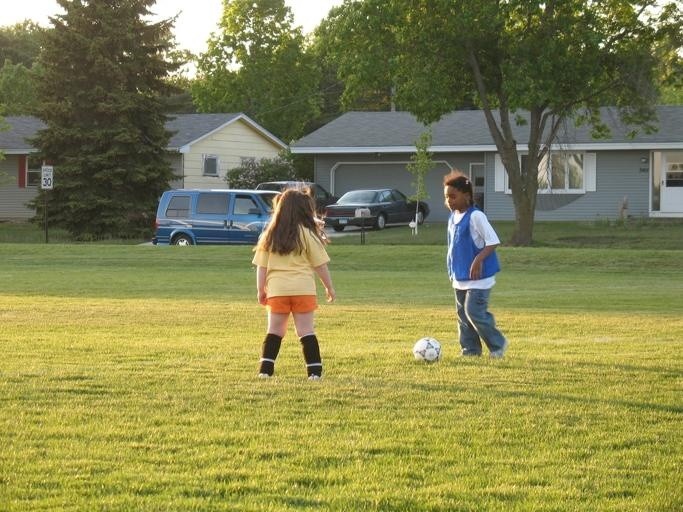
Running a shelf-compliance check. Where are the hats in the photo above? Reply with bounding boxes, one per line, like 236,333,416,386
322,189,430,231
473,168,483,209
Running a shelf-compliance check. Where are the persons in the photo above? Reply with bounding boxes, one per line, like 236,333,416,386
251,189,335,381
443,171,509,357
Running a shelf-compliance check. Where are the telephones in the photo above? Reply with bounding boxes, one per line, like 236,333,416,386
41,165,53,190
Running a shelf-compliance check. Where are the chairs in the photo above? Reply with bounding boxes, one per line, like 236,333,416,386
488,338,509,360
455,350,482,359
256,357,276,380
305,361,323,381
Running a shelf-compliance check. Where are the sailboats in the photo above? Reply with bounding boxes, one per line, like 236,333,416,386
413,337,441,361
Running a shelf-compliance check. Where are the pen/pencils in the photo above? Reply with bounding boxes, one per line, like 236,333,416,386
150,189,285,245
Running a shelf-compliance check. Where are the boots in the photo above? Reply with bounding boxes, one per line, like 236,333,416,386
255,182,346,232
